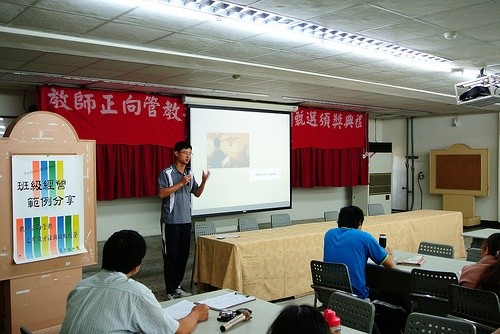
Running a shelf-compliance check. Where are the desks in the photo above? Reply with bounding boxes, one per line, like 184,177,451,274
193,210,466,302
160,288,367,334
462,228,500,239
367,250,478,281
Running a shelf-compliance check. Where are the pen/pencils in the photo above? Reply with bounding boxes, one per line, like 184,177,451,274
183,172,186,176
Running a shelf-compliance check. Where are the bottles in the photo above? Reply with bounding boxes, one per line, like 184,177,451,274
323,309,341,334
379,234,387,249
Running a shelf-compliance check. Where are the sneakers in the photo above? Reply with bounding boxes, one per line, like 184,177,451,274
173,289,193,296
167,294,183,299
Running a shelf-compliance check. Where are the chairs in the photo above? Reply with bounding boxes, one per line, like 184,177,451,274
238,219,260,231
369,204,384,215
190,222,216,289
325,211,338,221
271,214,290,228
311,240,500,334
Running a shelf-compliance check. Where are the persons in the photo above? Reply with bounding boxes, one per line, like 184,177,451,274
459,233,500,292
323,206,394,299
158,141,210,301
212,137,228,168
58,229,209,334
266,304,340,334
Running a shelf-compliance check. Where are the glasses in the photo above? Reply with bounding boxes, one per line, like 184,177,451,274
178,151,193,156
